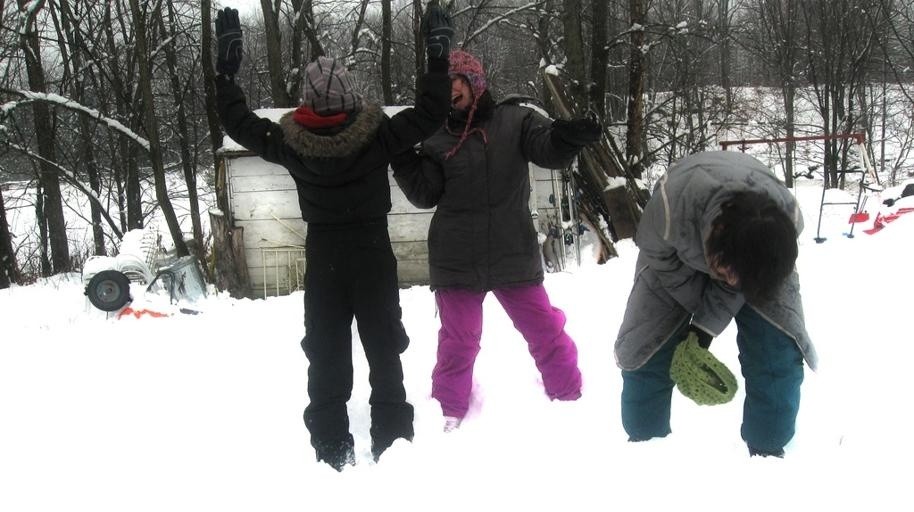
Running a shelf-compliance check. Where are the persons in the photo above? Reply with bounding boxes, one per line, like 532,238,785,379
388,50,602,434
212,0,454,471
611,149,814,459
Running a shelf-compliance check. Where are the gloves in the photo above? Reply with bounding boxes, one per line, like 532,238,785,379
550,117,602,152
214,7,243,76
421,1,454,61
680,323,712,348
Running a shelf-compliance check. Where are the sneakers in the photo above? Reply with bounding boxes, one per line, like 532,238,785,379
443,415,459,431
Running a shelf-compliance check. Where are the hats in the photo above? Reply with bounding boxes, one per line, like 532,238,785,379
448,50,487,100
668,331,737,405
301,55,363,116
